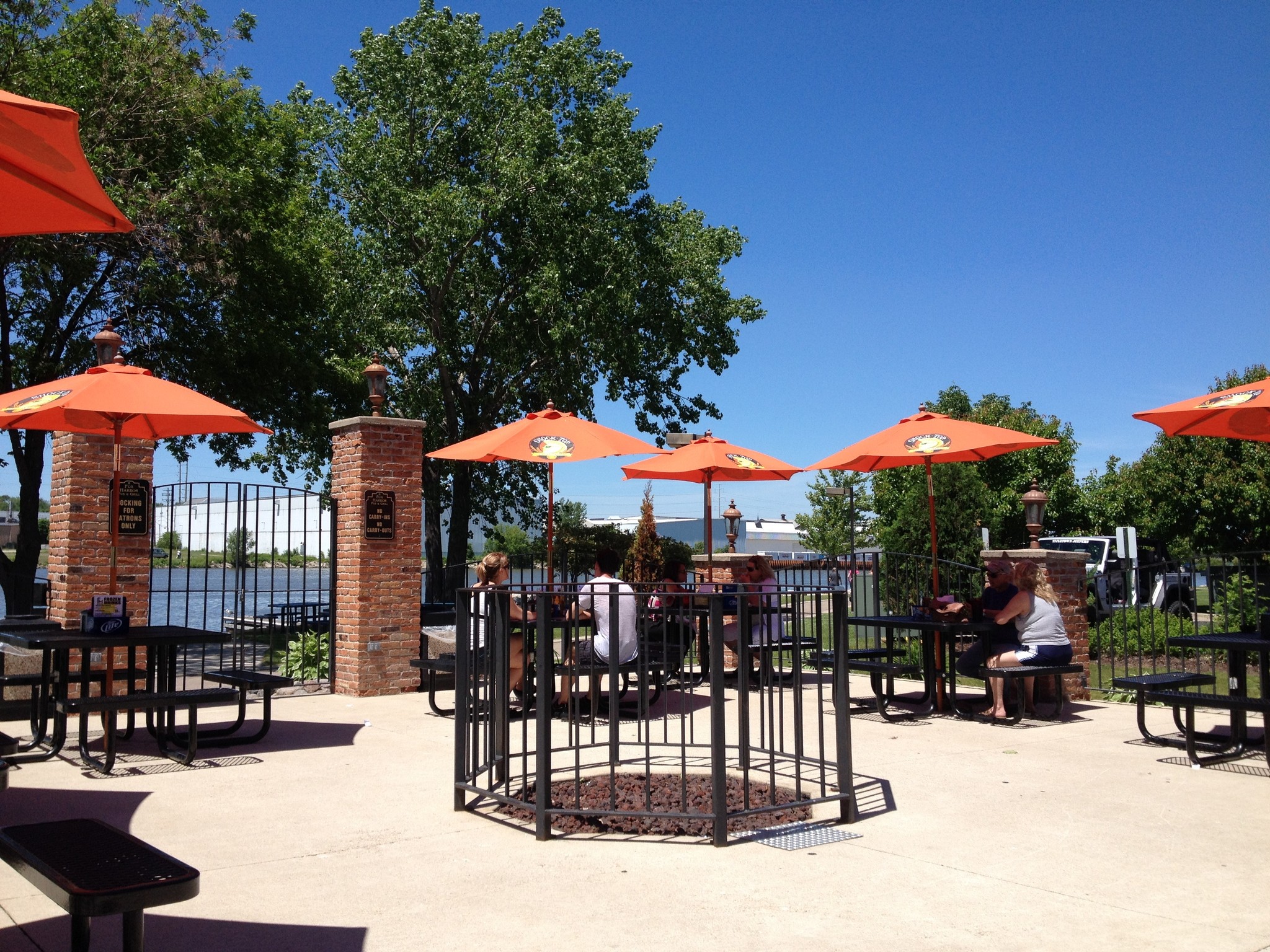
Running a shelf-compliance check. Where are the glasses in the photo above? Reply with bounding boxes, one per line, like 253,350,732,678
678,570,686,574
986,571,1004,578
746,565,756,572
503,564,509,569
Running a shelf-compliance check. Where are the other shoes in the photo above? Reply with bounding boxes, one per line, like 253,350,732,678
513,687,523,700
579,695,601,713
755,667,775,678
551,697,568,712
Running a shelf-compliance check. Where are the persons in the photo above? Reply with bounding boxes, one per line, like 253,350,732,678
723,555,785,679
954,560,1018,711
552,548,638,713
638,559,696,683
466,552,556,718
977,558,1073,720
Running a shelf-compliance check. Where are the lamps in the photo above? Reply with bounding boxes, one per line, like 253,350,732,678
359,350,392,418
90,316,126,365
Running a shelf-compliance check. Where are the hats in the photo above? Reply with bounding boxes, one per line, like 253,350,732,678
981,560,1009,573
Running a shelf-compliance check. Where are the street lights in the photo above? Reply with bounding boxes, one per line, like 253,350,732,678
825,487,854,612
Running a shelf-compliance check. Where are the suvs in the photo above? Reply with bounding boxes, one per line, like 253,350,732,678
1037,535,1195,628
149,547,169,559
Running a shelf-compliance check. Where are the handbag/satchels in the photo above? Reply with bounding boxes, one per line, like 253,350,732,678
933,602,971,623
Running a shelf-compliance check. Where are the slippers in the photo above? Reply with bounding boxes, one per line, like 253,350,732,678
979,709,1007,719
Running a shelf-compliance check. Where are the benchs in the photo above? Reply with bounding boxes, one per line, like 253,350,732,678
0,814,204,952
0,666,152,690
809,648,908,716
199,665,295,750
1145,689,1270,766
976,661,1085,727
253,615,278,631
621,639,689,691
1112,671,1217,753
53,683,241,774
731,640,816,693
264,612,300,627
554,657,675,724
409,658,492,717
807,657,921,722
317,610,330,617
307,620,330,627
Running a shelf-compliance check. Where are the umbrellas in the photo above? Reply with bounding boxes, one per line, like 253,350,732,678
424,398,672,583
1133,375,1270,444
0,86,136,239
0,354,273,755
619,428,804,583
801,402,1060,711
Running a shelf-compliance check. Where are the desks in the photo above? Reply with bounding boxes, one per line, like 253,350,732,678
1166,630,1270,751
636,604,792,681
267,601,330,634
0,623,236,773
847,614,1016,723
510,616,591,709
